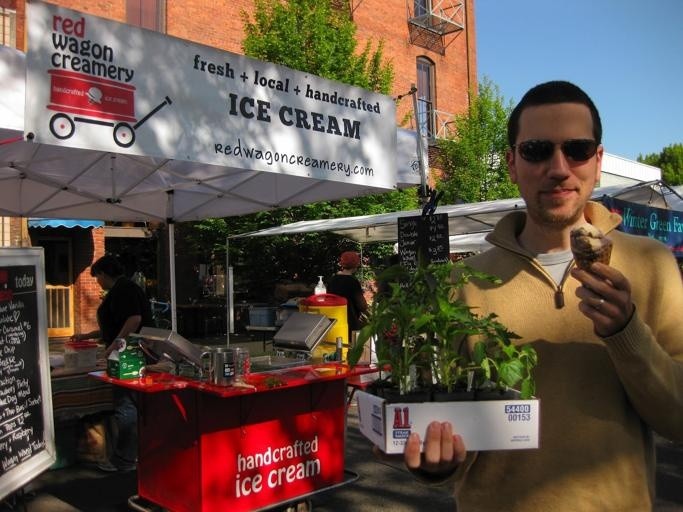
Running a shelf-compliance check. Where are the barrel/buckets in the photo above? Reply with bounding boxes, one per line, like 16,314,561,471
299,294,348,358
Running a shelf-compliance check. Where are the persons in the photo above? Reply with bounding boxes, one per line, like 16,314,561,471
66,253,154,474
399,79,683,511
325,250,369,407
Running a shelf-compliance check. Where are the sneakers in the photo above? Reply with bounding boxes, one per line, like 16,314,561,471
95,457,138,472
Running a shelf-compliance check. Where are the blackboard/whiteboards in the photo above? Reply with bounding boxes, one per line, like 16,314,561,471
0,246,57,503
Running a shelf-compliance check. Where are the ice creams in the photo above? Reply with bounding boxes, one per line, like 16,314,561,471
570,223,612,288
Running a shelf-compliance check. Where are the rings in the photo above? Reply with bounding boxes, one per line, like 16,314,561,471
595,298,606,310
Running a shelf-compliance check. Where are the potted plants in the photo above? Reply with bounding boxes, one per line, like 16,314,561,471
343,242,543,404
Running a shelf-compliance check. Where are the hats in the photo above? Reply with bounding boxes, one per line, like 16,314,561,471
340,252,360,267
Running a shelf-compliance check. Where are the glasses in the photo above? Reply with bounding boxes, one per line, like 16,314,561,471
510,136,599,162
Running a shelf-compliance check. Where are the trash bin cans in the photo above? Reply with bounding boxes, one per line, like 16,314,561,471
249,307,278,327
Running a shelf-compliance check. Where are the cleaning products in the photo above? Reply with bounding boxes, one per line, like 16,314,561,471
315,275,326,293
